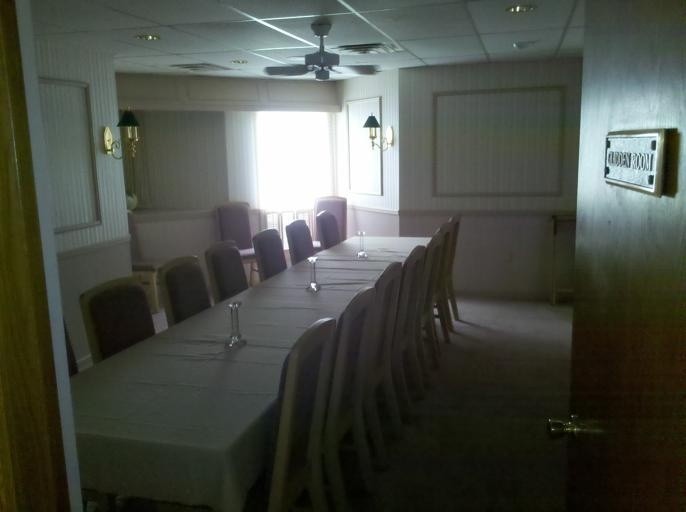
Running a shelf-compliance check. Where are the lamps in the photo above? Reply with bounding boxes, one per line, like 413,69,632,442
363,113,394,151
103,104,141,161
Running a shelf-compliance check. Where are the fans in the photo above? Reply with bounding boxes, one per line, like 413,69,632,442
264,22,381,79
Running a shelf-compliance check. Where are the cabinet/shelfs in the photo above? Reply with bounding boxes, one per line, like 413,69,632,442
549,214,576,305
